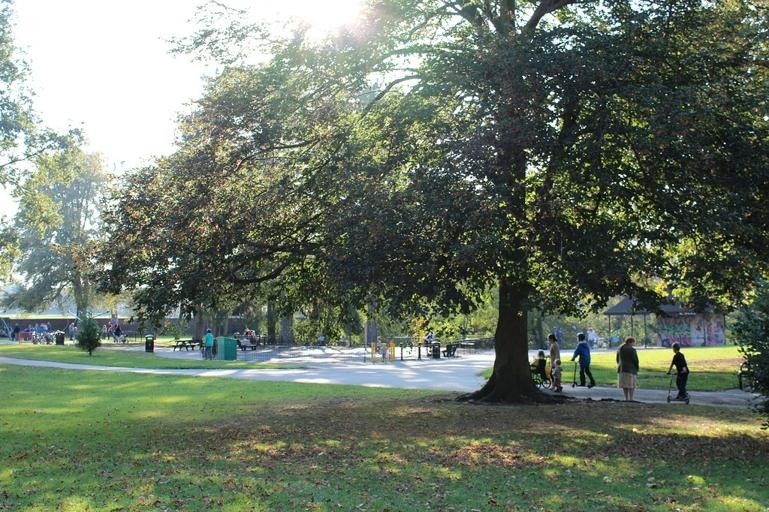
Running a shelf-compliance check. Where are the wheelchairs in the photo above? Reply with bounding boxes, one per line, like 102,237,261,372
528,364,551,389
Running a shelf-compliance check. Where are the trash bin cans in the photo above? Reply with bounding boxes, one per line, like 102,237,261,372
432,342,440,358
55,331,65,345
145,335,154,352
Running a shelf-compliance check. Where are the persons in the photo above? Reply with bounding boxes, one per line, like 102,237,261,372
570,332,594,388
530,351,544,366
550,358,563,391
615,336,640,400
423,329,433,357
545,333,561,392
667,341,689,399
9,317,262,359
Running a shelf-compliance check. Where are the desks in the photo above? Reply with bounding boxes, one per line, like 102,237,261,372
176,341,193,351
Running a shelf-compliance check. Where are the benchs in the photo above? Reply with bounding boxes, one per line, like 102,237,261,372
183,345,196,351
240,336,257,351
442,345,457,357
738,354,757,390
172,345,186,351
